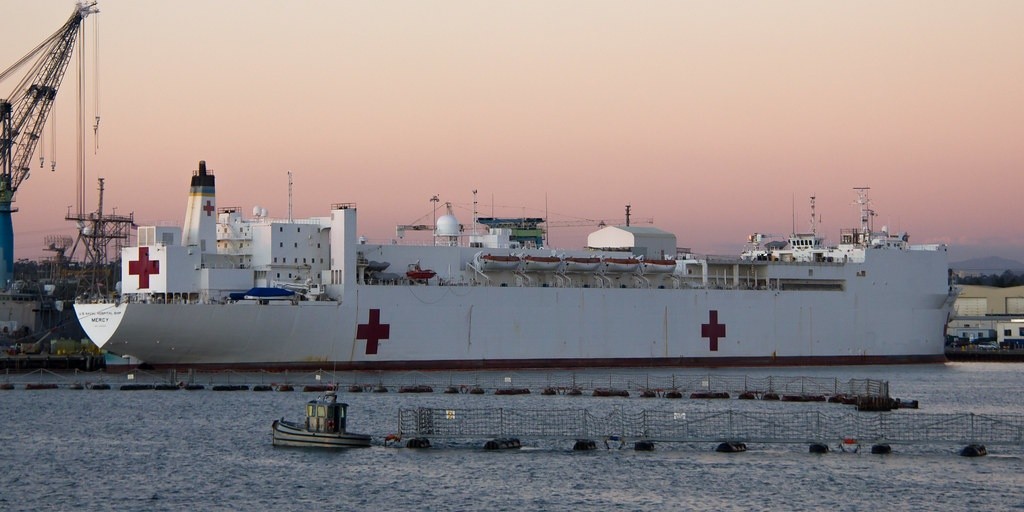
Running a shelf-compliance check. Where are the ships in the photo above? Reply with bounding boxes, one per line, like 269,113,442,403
72,159,962,374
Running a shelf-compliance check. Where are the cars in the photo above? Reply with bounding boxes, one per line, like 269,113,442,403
946,334,998,350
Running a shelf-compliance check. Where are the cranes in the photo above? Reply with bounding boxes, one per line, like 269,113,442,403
0,1,103,293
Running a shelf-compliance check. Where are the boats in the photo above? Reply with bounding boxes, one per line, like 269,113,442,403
642,259,677,273
481,253,520,270
603,257,640,272
522,255,560,271
406,269,437,279
272,383,372,448
563,256,601,271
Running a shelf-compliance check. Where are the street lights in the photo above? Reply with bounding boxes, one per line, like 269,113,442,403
430,196,439,247
